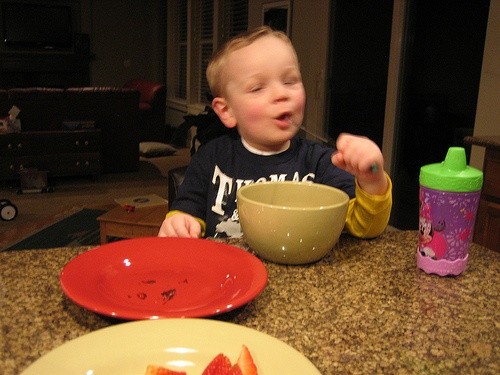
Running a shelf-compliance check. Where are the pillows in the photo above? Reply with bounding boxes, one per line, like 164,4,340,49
139,142,177,157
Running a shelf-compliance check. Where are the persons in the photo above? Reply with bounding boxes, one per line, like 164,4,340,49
157,25,392,239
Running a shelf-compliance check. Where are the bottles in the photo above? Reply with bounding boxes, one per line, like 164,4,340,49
416,147,483,277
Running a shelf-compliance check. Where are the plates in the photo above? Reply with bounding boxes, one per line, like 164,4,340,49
59,236,268,321
19,318,321,375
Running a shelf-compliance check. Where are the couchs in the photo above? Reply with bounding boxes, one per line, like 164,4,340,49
0,79,167,174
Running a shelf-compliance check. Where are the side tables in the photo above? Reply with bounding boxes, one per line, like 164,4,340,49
97,206,167,245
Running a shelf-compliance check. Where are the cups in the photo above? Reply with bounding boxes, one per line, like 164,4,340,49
0,198,18,221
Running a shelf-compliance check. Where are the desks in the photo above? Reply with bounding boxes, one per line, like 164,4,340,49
0,45,90,85
0,231,500,375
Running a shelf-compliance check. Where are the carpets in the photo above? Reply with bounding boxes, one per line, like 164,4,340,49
0,208,125,251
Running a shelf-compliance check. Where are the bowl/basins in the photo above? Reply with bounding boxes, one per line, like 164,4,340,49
236,181,351,266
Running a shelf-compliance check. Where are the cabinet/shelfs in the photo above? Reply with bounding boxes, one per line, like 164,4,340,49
472,144,500,255
0,128,104,185
